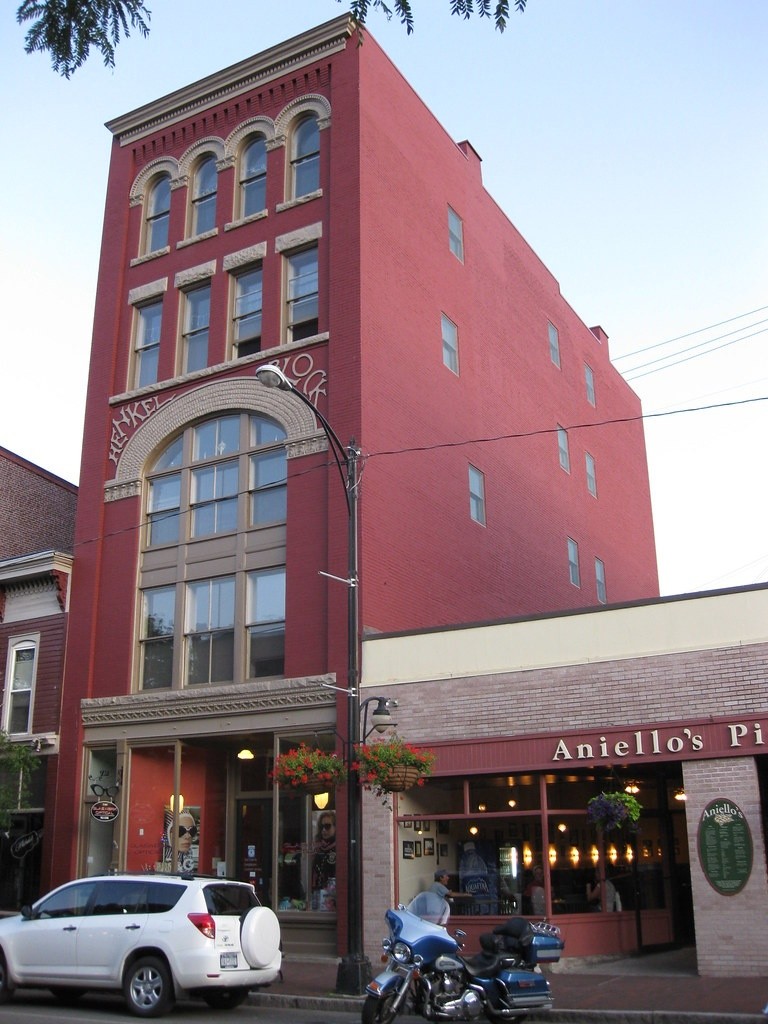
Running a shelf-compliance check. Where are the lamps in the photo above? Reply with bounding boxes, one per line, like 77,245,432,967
624,782,640,793
314,792,329,809
237,749,254,759
674,789,685,801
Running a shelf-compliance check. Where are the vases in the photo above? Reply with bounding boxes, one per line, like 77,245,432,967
382,763,419,792
302,771,331,795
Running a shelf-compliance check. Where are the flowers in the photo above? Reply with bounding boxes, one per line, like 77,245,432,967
588,791,643,847
351,730,438,811
267,742,346,795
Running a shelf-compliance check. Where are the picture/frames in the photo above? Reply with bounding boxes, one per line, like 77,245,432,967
424,837,434,856
403,840,414,859
423,814,430,831
439,820,449,834
415,840,421,857
404,814,412,828
439,844,448,856
414,813,421,831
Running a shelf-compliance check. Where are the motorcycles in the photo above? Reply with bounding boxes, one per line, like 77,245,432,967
361,892,566,1024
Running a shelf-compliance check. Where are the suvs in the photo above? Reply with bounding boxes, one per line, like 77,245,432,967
1,872,283,1018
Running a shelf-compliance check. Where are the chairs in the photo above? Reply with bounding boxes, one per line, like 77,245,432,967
453,894,476,915
497,891,517,915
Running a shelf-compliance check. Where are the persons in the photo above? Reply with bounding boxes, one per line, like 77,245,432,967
311,812,336,893
164,808,195,873
585,869,615,912
427,867,473,915
523,864,554,899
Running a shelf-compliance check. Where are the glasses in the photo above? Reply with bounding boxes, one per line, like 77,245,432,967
321,823,333,831
177,825,197,838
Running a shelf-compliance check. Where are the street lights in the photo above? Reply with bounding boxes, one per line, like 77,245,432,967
254,360,372,997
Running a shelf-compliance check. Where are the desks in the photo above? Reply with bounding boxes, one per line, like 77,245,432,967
475,899,498,916
556,894,586,913
